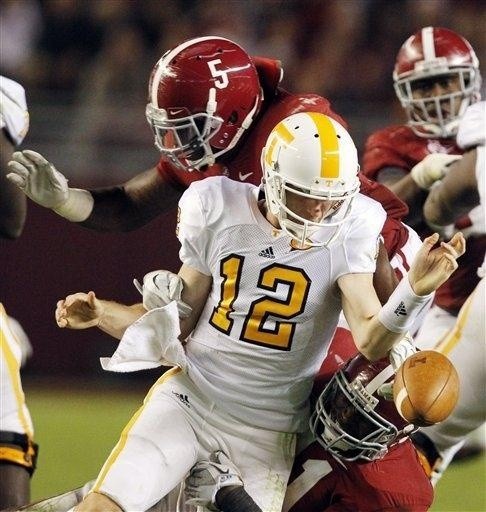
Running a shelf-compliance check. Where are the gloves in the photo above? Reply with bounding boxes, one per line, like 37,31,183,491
6,150,94,223
134,270,192,319
185,450,244,512
412,154,462,189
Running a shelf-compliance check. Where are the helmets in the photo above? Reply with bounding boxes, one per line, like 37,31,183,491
392,27,482,112
260,111,361,216
336,344,421,444
145,36,265,150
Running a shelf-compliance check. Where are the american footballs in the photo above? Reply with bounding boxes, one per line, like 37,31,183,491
393,351,461,426
